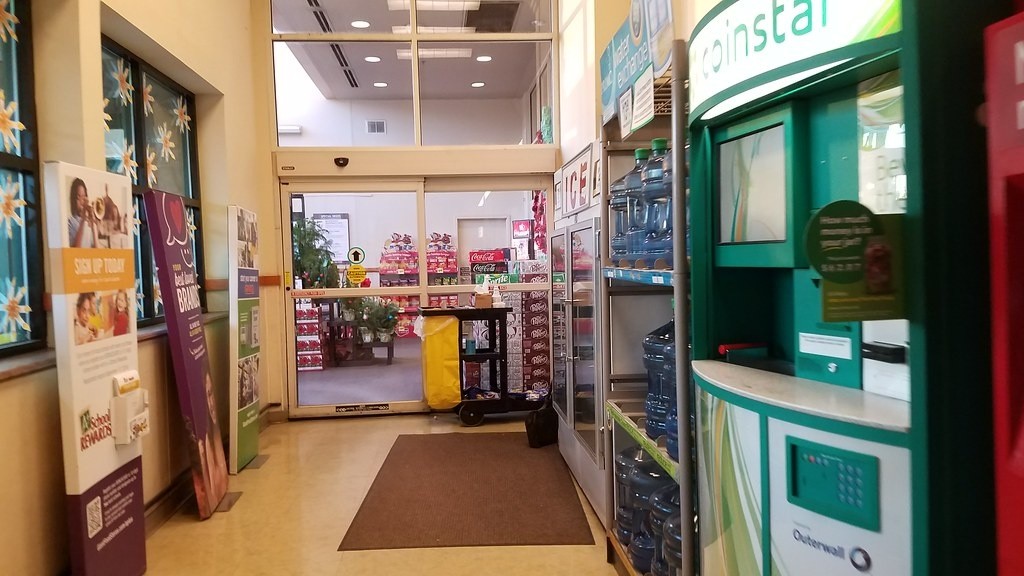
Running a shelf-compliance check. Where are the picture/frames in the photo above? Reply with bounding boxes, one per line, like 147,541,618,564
561,142,592,218
513,220,530,239
593,159,601,198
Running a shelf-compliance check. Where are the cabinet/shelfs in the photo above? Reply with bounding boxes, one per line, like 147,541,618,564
380,269,458,312
596,39,695,576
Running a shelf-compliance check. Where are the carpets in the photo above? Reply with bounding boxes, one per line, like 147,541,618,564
336,431,597,551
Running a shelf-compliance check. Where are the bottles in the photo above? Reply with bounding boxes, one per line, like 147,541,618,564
531,131,546,144
615,299,700,576
610,126,690,269
492,286,501,304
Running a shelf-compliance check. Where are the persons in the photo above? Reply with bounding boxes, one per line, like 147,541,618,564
114,290,128,336
110,207,124,233
68,178,100,248
202,359,228,501
75,292,98,346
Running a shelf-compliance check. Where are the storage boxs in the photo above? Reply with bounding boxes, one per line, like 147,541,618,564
382,278,418,287
381,296,419,306
381,249,457,268
395,317,418,338
435,277,457,285
470,259,550,391
295,279,327,371
429,295,457,306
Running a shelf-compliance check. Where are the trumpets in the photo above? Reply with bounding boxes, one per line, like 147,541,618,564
83,196,106,223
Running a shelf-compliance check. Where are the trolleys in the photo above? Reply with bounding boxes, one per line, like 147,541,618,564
417,306,548,426
